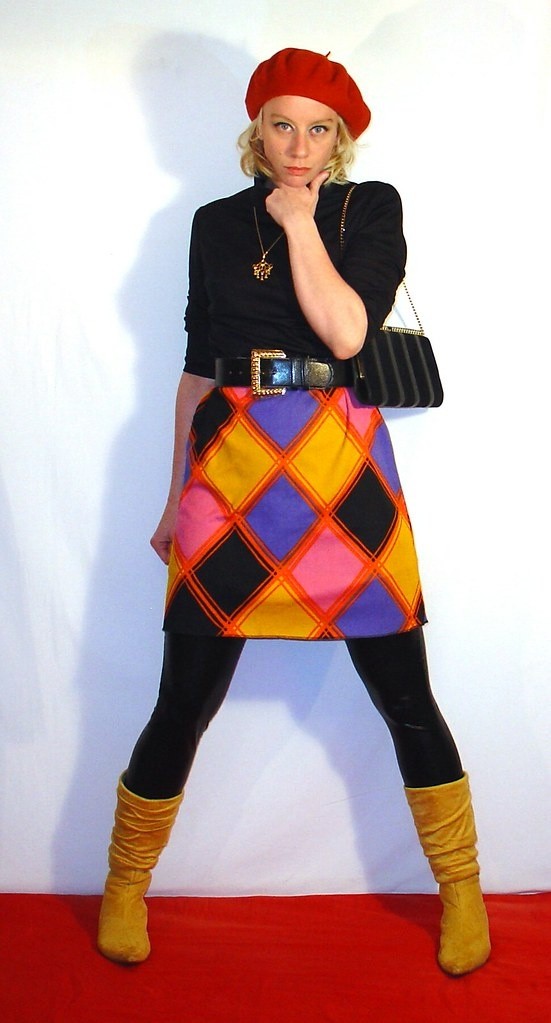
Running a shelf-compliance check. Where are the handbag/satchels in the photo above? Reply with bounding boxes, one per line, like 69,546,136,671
340,183,443,409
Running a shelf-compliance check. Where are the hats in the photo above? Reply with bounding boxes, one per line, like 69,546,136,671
245,47,371,141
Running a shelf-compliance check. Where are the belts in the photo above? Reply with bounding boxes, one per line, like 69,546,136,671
214,349,354,397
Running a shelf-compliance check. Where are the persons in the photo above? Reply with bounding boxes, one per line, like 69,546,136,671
97,47,490,976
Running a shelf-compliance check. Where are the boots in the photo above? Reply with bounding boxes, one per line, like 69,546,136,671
405,772,491,977
96,768,185,965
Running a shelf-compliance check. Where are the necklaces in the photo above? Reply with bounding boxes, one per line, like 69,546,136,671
252,205,284,281
